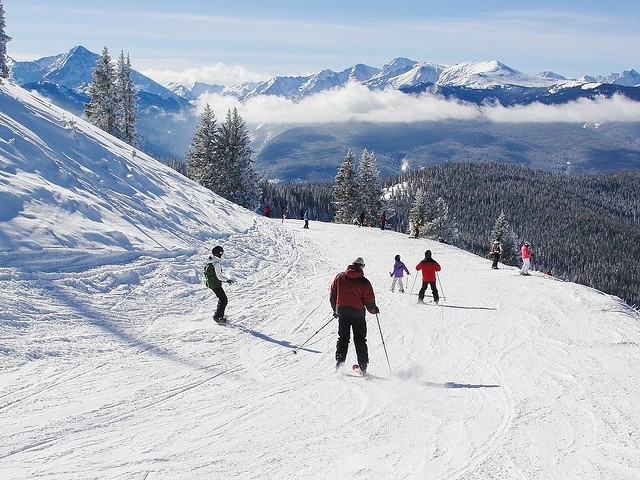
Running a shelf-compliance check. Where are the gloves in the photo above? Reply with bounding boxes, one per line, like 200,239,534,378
407,272,409,275
227,278,234,286
391,274,393,277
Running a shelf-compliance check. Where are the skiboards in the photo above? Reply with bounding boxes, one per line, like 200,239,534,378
388,288,405,293
335,365,370,380
418,299,439,307
510,274,534,277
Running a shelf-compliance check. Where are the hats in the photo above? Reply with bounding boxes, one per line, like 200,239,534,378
395,255,400,260
212,246,224,254
353,257,365,268
496,237,500,241
525,241,531,246
425,250,431,257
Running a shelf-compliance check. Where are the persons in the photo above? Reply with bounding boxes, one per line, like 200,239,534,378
416,250,442,306
390,255,410,293
203,246,234,325
359,211,367,226
329,257,379,375
303,210,310,229
491,237,502,269
264,206,271,218
518,241,534,275
413,218,423,239
381,212,388,230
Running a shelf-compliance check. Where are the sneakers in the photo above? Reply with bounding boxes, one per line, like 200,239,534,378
520,272,523,274
335,360,345,369
360,363,367,371
523,273,531,276
400,289,404,292
390,288,395,291
434,300,439,305
494,267,499,269
417,299,424,303
213,317,226,323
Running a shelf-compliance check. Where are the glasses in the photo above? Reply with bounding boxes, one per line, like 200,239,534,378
355,263,365,267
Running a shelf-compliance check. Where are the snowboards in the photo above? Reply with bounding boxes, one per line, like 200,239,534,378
213,315,227,325
